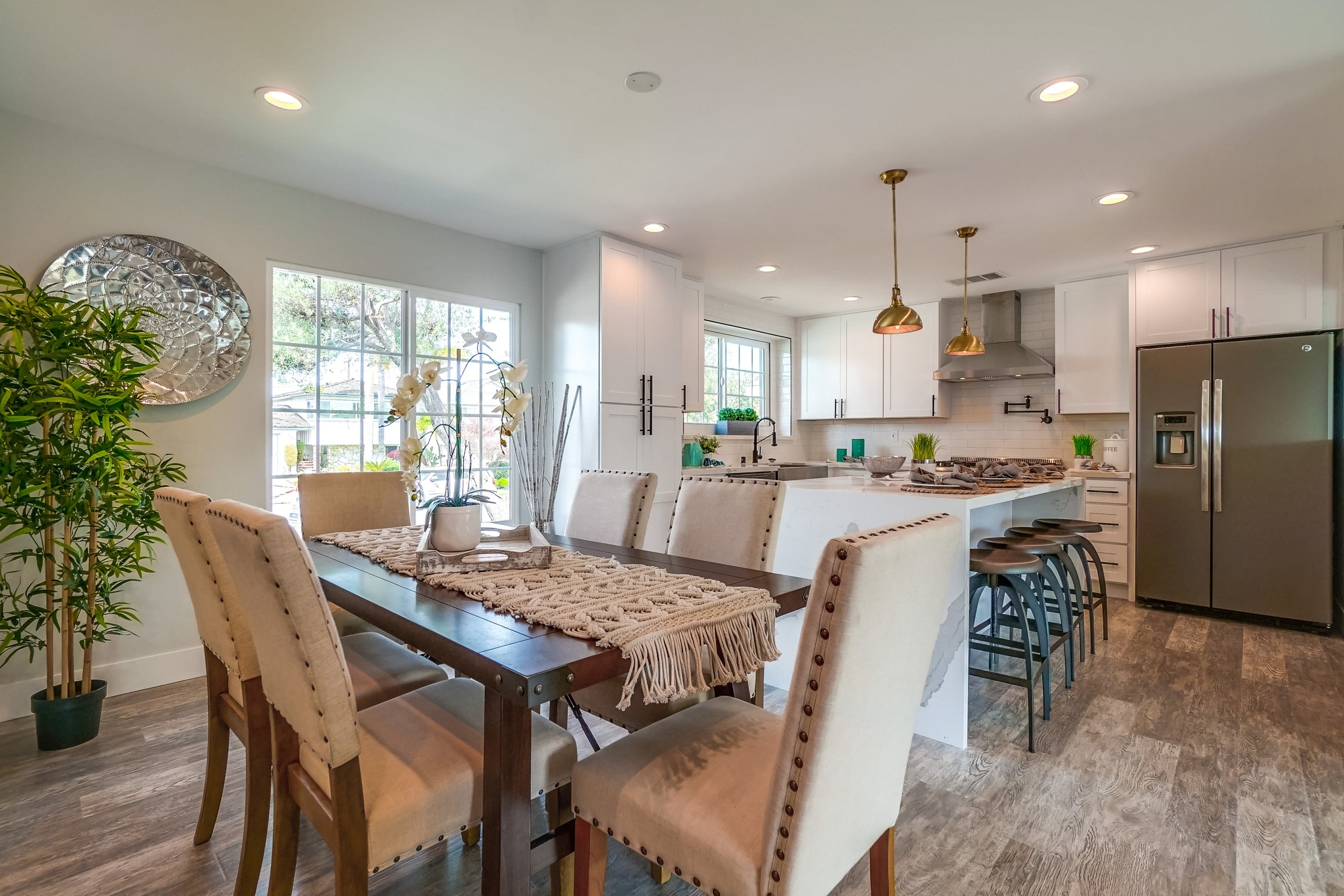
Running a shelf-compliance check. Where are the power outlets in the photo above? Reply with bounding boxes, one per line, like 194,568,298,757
891,430,898,442
1111,430,1122,439
796,431,802,442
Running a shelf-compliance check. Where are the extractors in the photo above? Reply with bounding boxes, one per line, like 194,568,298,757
933,290,1055,382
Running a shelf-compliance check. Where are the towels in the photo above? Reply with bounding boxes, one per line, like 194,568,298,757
704,458,725,466
842,455,861,462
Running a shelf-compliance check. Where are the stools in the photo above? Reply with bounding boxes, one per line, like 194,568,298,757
970,518,1109,754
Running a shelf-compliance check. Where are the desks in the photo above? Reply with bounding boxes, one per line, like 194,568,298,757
305,528,813,896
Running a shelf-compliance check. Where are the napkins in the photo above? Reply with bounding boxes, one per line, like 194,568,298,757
909,459,1069,491
842,456,861,462
1081,460,1117,472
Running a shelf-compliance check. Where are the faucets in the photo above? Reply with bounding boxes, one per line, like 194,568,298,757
752,416,778,463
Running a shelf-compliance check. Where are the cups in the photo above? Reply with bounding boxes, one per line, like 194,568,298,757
836,449,847,462
852,439,864,459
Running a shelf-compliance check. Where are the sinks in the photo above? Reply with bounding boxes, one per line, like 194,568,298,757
754,462,828,481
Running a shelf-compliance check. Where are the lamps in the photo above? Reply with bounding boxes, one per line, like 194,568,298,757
873,170,924,337
944,227,987,356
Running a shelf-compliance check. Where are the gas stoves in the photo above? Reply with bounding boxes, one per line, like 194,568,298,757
935,456,1066,472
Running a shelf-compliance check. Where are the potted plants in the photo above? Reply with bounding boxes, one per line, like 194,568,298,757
716,408,760,436
694,433,722,459
1065,434,1099,469
0,261,188,751
904,432,947,474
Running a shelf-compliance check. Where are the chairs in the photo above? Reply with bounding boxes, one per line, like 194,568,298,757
154,468,964,896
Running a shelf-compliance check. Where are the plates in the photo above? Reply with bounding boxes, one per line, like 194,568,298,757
974,477,1014,483
910,482,964,489
1044,471,1058,476
1020,476,1031,480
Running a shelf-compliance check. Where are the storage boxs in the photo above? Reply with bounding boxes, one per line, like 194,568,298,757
416,524,551,575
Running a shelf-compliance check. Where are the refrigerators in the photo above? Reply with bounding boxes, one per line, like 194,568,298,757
1135,332,1336,637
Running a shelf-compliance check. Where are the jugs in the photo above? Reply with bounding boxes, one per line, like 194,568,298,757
682,442,703,466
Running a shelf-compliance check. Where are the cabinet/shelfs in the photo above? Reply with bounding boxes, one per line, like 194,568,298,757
798,301,951,421
1136,232,1324,347
1084,477,1137,603
1054,273,1128,415
540,234,705,505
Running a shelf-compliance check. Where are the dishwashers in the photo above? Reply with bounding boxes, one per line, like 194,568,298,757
726,471,777,480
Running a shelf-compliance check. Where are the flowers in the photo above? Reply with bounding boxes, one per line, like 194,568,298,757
378,325,532,533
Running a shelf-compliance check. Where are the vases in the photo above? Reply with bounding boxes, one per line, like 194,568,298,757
530,519,556,535
430,502,481,552
